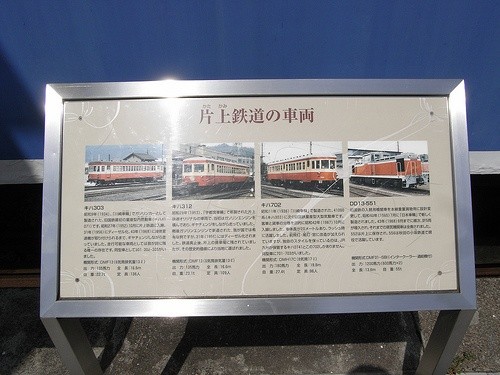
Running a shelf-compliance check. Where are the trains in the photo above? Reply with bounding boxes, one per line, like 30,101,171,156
350,158,423,190
265,154,337,188
181,157,253,190
87,161,165,186
